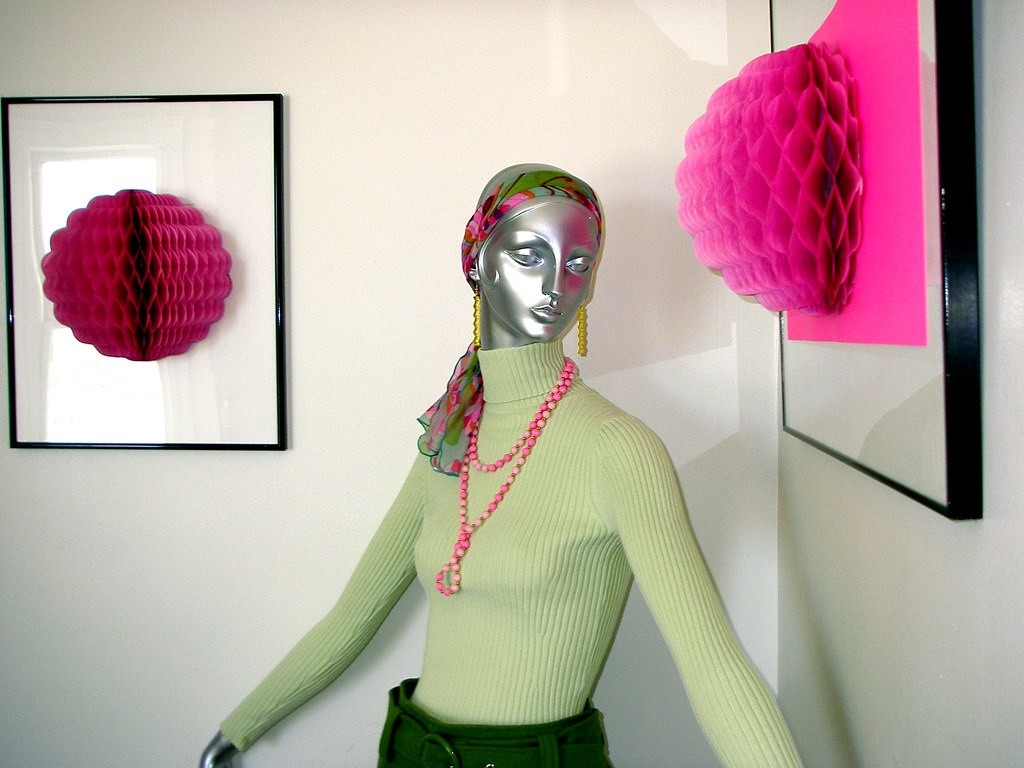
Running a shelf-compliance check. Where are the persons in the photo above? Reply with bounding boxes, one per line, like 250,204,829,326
200,163,807,768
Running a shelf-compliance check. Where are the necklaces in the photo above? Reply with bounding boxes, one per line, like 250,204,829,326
435,357,579,595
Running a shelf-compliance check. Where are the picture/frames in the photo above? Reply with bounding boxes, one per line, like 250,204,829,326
0,93,288,452
677,0,985,524
